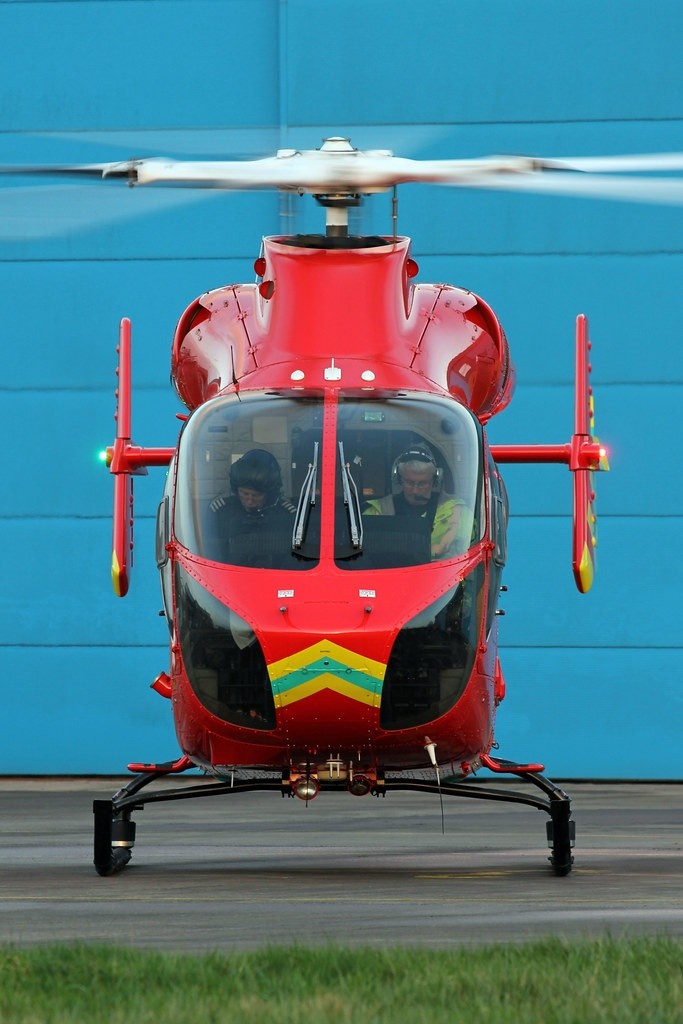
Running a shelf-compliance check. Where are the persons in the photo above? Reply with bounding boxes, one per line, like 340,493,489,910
355,447,480,720
191,447,303,723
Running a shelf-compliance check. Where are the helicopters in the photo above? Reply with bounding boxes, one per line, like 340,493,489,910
2,132,681,879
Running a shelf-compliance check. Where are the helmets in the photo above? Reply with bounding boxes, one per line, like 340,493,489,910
231,450,280,505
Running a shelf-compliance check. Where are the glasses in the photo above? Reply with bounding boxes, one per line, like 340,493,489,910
401,480,431,489
